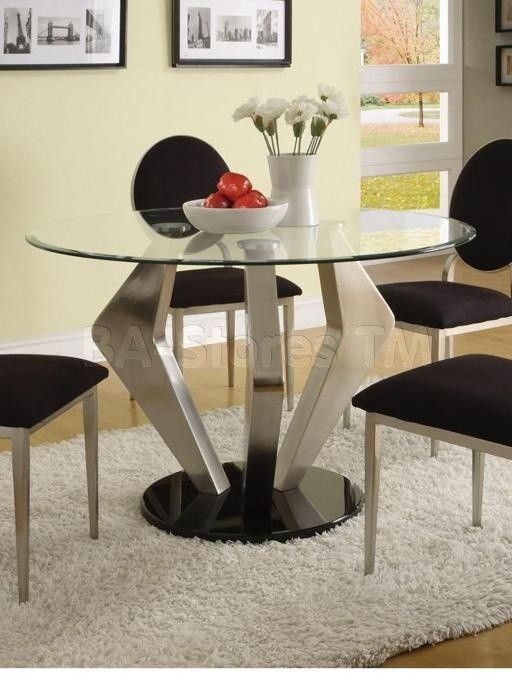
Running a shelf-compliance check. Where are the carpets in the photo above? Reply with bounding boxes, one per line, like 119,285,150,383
2,395,510,667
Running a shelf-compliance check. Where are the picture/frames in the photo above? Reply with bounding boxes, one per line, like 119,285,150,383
494,1,512,87
0,0,294,72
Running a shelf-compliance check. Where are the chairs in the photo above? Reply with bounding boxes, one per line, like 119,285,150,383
129,132,511,461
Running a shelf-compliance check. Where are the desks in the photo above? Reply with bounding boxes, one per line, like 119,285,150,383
22,205,479,544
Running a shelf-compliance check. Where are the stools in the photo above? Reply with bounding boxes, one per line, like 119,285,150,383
351,352,511,574
0,351,108,604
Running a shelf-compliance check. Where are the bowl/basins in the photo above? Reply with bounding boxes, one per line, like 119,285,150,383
182,198,289,235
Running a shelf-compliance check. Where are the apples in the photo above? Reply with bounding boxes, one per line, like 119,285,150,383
234,190,265,209
216,173,252,202
205,192,231,207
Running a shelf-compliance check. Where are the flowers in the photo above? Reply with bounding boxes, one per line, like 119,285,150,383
231,80,351,155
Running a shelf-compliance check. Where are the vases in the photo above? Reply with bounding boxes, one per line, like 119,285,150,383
265,154,320,230
